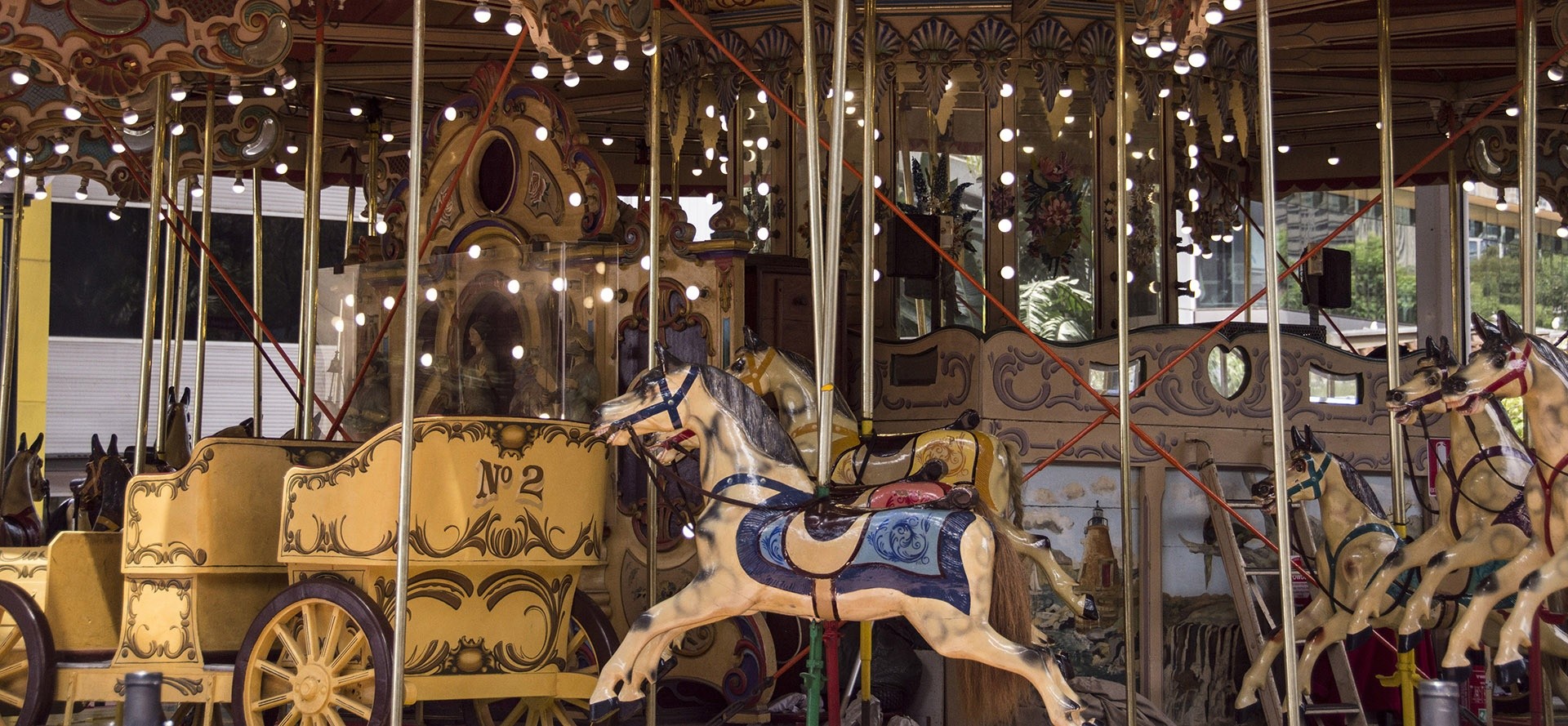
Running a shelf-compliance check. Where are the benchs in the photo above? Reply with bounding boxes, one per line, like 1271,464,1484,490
116,418,629,674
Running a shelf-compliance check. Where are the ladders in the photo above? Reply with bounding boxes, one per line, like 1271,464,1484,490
1198,443,1368,726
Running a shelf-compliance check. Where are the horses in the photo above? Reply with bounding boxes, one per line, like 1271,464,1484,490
591,326,1098,726
1235,311,1568,725
1,387,254,547
